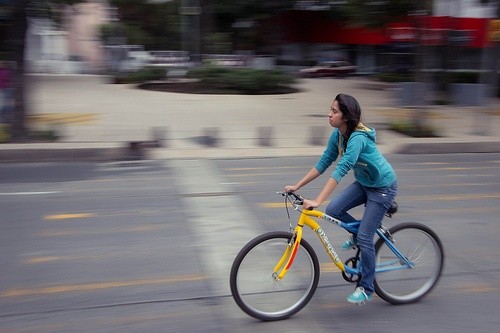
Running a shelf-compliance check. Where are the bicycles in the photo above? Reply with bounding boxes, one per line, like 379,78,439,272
229,186,444,321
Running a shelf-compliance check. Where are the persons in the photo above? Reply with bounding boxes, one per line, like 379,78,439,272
283,93,396,303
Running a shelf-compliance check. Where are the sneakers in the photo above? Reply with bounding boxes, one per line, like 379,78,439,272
346,286,374,302
341,234,357,250
